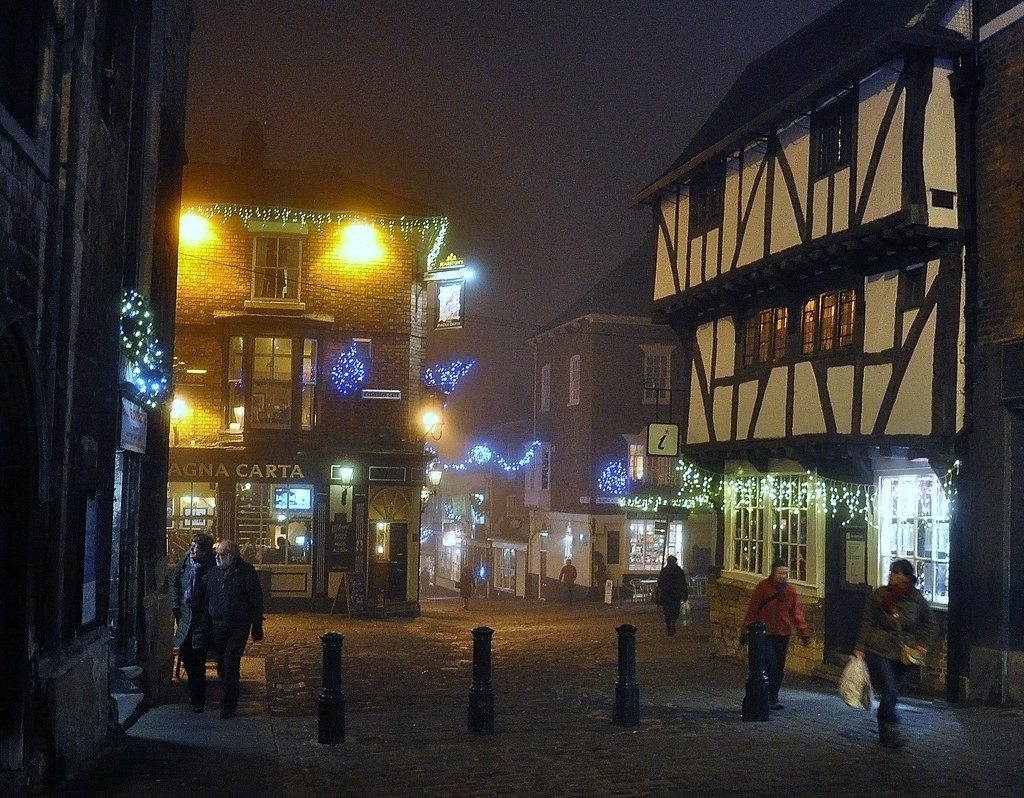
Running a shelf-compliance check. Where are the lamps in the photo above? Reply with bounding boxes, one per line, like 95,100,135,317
340,454,355,506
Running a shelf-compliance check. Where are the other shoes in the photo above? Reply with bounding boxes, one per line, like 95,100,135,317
770,703,784,711
879,728,907,745
193,706,204,713
220,708,235,719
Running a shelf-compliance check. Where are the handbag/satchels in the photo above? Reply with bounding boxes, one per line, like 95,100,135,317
901,646,924,667
860,667,872,710
840,656,865,709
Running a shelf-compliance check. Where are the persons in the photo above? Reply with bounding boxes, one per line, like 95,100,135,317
168,533,217,713
558,559,578,604
738,561,812,713
851,560,937,748
237,537,304,564
421,566,431,595
200,539,264,720
252,398,289,424
459,566,476,610
656,556,688,636
276,489,298,507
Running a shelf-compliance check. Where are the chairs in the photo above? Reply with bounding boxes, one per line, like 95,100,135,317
630,577,658,603
689,574,709,598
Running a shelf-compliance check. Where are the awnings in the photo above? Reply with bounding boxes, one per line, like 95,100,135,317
213,309,336,327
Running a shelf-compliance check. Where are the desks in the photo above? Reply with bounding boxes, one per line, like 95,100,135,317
642,580,657,600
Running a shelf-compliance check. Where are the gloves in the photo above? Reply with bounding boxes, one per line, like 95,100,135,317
251,624,264,642
173,609,182,626
739,634,748,646
803,637,810,647
